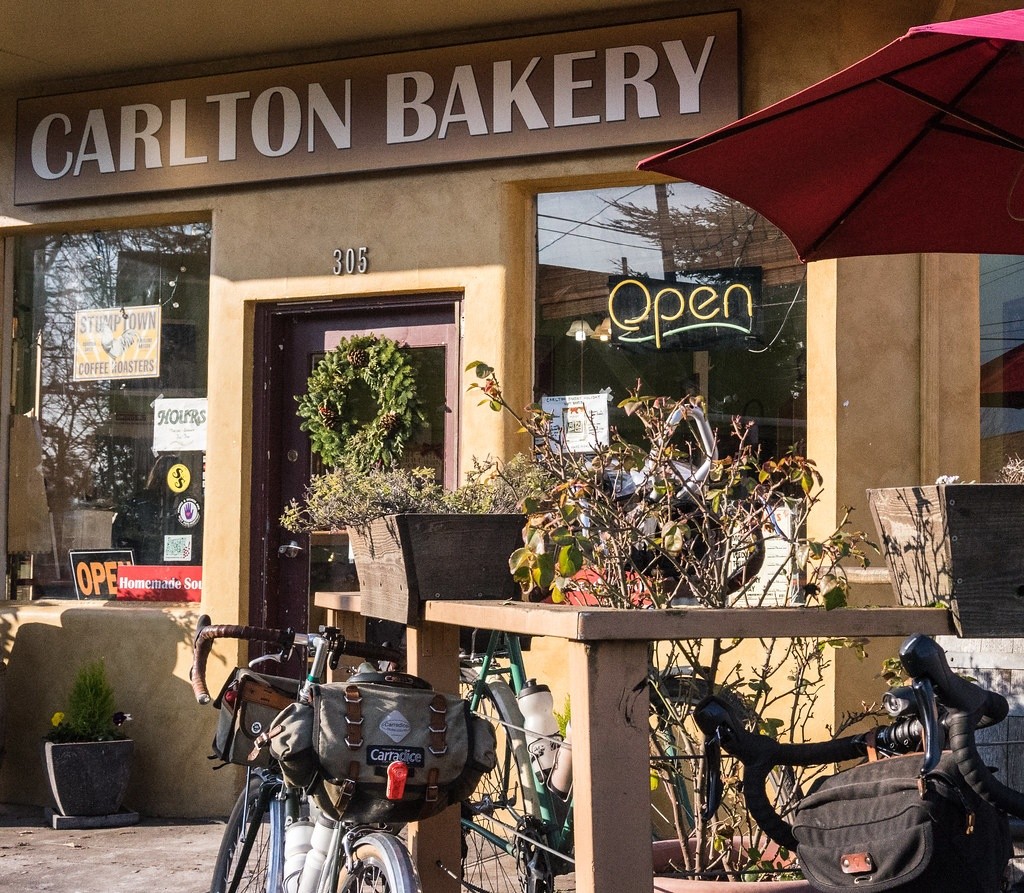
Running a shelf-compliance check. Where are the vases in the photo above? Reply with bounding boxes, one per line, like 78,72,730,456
41,732,141,831
864,482,1024,638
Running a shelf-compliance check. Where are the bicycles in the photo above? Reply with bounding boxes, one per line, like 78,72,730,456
187,612,423,893
458,628,1023,893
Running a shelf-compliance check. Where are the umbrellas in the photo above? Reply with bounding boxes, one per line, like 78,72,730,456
635,7,1024,268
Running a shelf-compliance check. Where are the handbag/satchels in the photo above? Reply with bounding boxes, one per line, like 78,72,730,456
790,749,1015,893
247,681,498,823
207,666,305,770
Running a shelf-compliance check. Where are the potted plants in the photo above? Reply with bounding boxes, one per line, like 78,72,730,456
280,449,593,628
463,352,952,893
40,655,141,738
